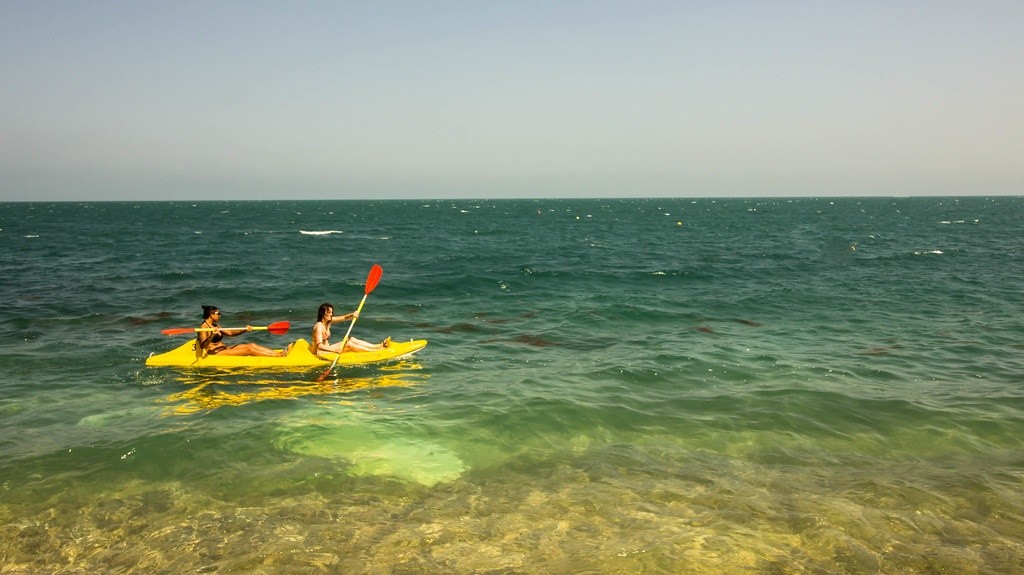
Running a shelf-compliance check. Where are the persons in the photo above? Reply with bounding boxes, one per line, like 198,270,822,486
199,305,293,357
313,304,391,355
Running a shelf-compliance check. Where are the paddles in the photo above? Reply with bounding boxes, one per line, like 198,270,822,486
161,320,290,335
315,263,384,383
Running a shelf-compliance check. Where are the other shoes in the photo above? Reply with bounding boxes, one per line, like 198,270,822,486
280,343,294,357
382,336,391,349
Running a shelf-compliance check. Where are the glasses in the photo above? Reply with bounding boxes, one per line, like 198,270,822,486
212,312,221,316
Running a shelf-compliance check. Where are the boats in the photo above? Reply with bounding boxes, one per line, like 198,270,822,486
144,337,428,369
